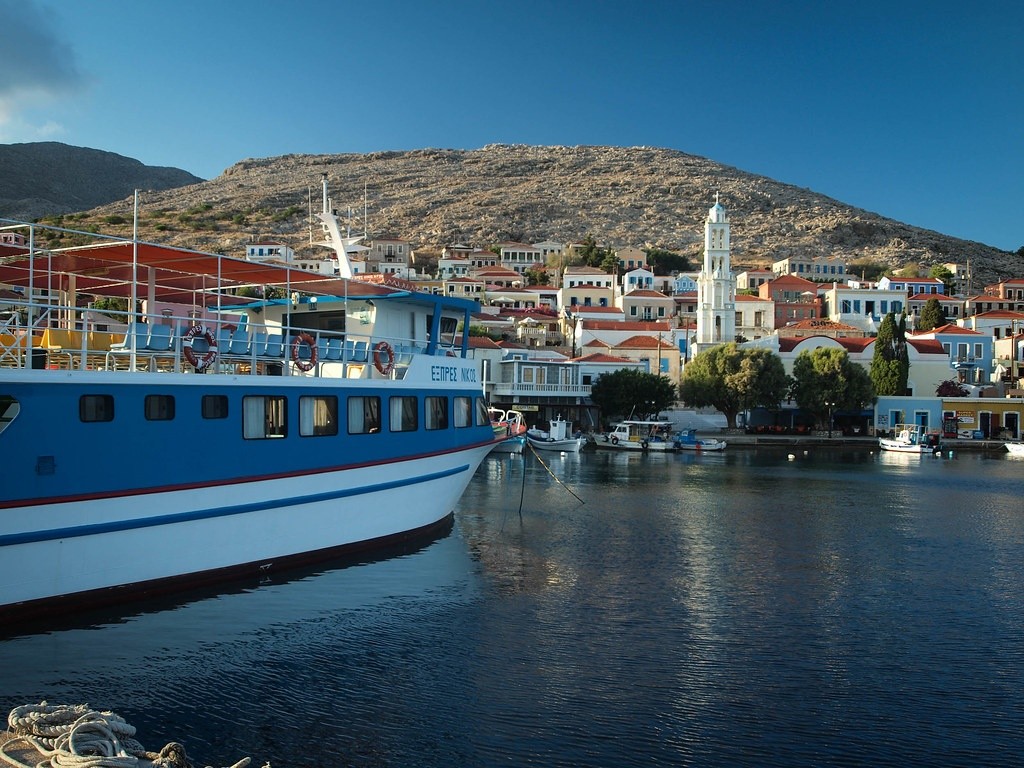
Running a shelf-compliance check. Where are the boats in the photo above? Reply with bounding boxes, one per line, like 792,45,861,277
920,433,942,452
491,435,526,454
672,428,727,450
1004,442,1024,454
1,188,528,606
593,421,683,450
878,424,933,452
526,413,587,452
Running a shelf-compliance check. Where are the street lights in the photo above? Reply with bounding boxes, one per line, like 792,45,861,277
645,398,655,421
824,399,835,438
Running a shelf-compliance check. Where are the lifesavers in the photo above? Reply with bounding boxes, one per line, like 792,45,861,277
292,333,318,372
612,437,619,445
183,323,219,369
642,441,648,448
373,340,396,376
